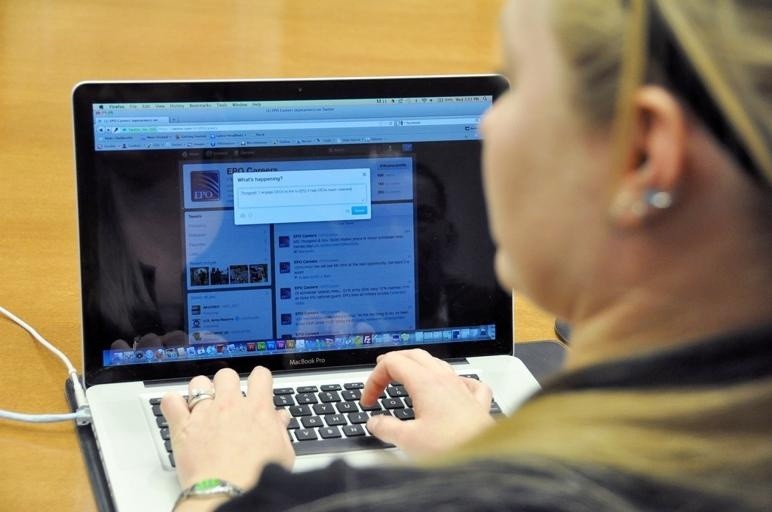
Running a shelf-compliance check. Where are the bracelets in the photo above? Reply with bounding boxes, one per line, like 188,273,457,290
171,477,244,511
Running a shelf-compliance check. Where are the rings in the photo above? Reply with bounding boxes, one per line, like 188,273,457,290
187,390,214,410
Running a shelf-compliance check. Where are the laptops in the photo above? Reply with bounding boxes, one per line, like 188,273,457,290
72,73,547,512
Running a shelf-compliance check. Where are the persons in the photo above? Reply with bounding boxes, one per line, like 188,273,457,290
154,1,771,511
413,163,511,357
